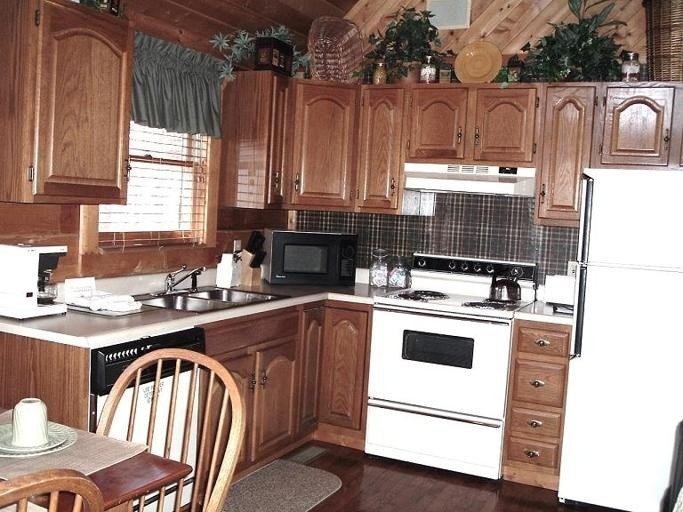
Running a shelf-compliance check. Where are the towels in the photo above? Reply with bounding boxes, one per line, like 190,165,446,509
65,288,141,313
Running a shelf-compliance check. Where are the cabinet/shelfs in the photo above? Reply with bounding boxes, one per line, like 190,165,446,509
535,84,597,231
201,298,324,491
501,318,574,492
220,68,283,210
588,80,682,168
404,82,543,169
283,80,359,211
316,297,373,448
354,83,436,218
0,0,135,204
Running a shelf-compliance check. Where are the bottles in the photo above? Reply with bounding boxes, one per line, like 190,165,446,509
387,254,411,288
620,51,641,82
420,56,436,82
368,251,388,287
372,61,388,84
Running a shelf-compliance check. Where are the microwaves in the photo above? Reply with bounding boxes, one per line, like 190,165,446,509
258,228,358,287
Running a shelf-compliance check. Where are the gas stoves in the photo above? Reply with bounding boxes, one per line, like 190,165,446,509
374,253,537,319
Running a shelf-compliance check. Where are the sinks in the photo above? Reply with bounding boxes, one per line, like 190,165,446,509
185,285,290,308
136,293,236,315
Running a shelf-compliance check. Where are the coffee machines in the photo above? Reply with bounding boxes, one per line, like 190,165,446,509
0,243,69,322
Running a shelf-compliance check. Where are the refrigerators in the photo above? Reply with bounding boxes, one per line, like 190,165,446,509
556,168,683,512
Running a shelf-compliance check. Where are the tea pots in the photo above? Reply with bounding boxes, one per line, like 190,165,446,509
487,279,521,307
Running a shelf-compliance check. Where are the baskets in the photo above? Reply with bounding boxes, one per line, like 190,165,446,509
308,15,363,84
642,1,683,82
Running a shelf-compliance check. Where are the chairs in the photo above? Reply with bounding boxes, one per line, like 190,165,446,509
96,348,247,512
0,467,105,512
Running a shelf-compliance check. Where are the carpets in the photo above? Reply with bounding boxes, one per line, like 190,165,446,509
221,459,343,512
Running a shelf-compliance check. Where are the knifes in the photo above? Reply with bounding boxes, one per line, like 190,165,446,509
244,230,268,268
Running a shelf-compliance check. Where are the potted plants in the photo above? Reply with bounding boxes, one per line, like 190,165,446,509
207,24,313,78
365,5,451,84
507,0,631,81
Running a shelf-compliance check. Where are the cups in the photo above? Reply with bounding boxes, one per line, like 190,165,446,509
11,396,49,449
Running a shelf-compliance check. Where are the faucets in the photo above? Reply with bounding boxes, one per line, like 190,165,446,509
164,263,206,295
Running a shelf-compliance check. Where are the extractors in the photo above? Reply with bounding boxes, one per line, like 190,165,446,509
402,162,537,198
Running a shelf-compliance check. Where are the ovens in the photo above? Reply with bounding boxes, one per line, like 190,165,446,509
362,307,512,481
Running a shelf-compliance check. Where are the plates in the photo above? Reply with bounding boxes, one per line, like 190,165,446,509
0,423,78,458
453,40,502,86
0,422,66,453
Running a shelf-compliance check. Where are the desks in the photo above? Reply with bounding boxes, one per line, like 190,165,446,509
0,408,192,512
